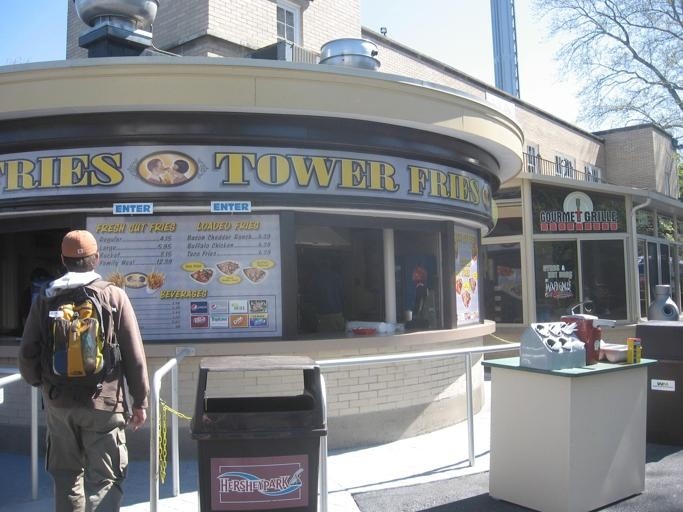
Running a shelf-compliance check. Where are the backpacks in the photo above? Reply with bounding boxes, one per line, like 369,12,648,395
40,280,123,390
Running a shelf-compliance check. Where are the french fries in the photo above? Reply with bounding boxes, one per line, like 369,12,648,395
147,271,166,290
109,271,126,288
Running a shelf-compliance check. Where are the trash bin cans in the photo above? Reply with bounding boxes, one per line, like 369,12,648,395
635,319,683,447
188,356,329,512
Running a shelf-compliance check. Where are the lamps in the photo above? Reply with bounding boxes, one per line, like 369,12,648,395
381,27,387,36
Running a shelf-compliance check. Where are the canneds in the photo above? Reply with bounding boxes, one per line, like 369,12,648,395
627,338,640,364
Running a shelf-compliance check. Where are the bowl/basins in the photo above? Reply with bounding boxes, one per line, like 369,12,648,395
352,327,376,335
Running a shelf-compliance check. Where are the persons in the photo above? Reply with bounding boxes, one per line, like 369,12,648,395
145,157,165,184
296,254,349,334
15,227,151,512
168,159,190,185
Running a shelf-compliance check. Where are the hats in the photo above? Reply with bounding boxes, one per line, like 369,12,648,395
61,230,98,258
